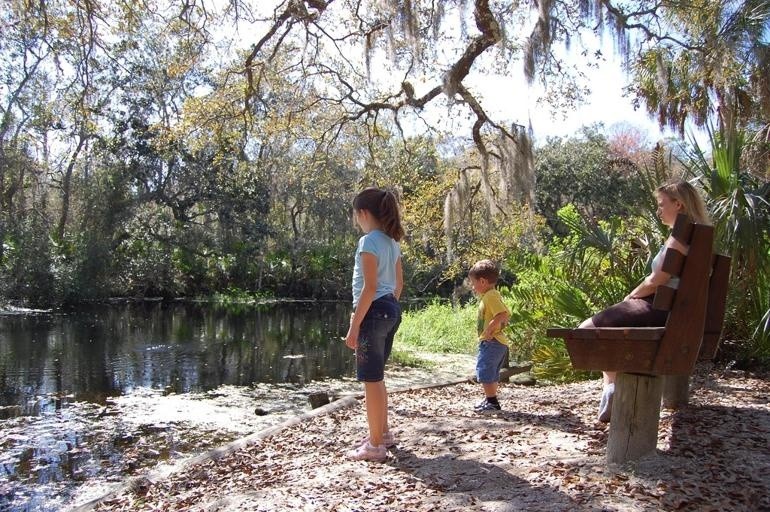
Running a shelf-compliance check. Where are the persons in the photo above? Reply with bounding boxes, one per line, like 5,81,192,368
468,258,510,414
342,188,406,461
578,178,711,422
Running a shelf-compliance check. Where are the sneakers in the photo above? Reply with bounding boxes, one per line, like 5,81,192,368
473,398,501,410
346,430,395,463
596,383,615,421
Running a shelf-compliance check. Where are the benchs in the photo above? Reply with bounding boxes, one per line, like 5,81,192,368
547,224,733,464
